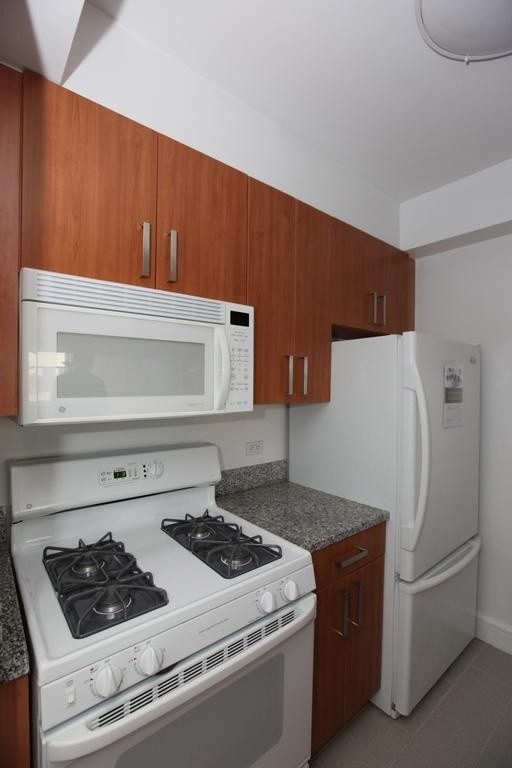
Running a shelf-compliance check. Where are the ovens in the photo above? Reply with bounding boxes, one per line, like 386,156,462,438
35,592,318,768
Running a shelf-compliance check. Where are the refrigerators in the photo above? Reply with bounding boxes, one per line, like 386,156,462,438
287,332,485,720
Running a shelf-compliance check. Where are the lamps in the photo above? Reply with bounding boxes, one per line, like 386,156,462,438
414,0,510,67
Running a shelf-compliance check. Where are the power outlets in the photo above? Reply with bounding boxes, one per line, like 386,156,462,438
245,440,265,457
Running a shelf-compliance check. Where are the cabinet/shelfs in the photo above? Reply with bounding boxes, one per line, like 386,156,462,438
0,54,23,412
243,172,333,408
310,523,391,757
333,211,416,337
0,674,31,768
22,66,247,306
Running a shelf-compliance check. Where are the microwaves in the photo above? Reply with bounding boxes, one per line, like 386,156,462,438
16,267,256,429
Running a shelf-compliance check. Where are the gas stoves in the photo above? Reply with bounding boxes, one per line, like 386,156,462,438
11,507,321,736
